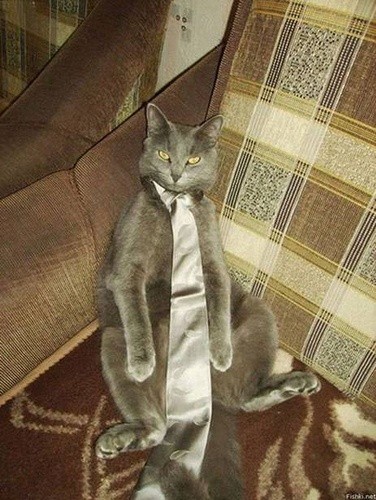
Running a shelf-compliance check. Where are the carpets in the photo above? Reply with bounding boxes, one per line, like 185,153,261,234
0,330,375,499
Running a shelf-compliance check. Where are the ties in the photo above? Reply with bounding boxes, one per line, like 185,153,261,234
131,178,213,500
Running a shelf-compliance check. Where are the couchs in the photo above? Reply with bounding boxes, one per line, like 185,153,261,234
2,2,375,420
4,0,171,191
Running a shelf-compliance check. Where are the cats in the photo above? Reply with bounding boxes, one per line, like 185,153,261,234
95,103,322,500
